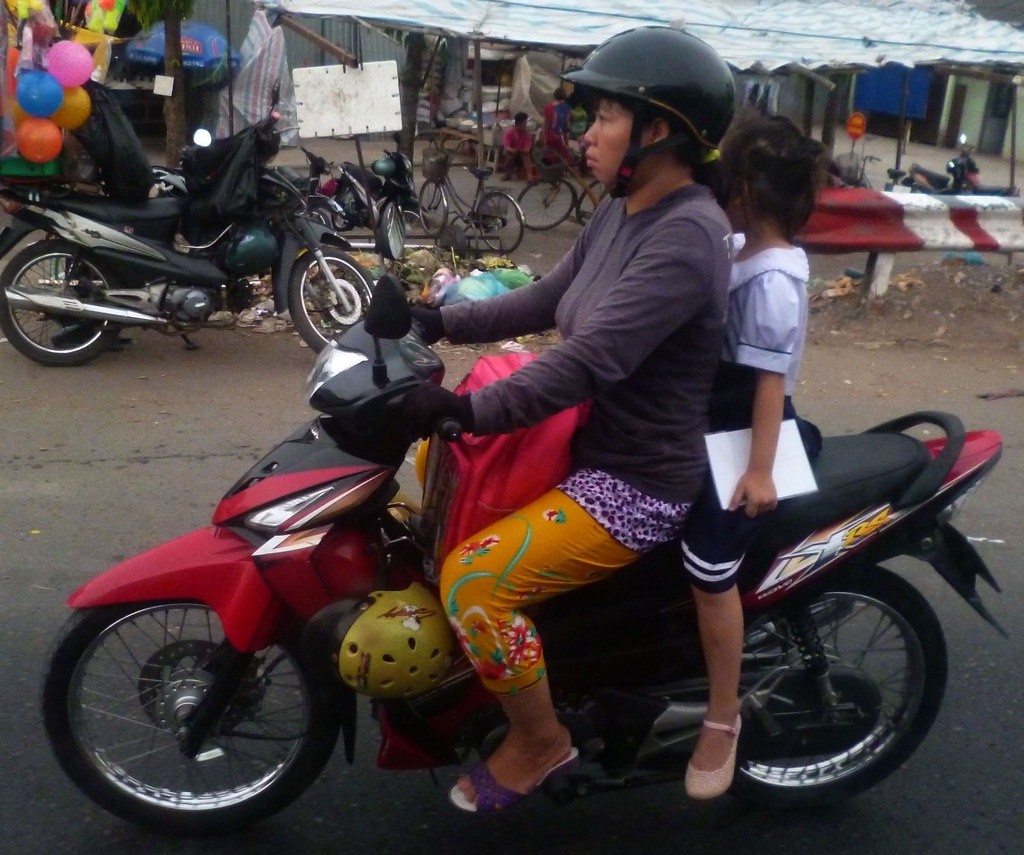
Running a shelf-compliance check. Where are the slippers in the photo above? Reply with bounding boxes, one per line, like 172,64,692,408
448,747,579,813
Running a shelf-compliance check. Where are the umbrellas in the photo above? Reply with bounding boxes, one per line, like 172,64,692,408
127,18,242,69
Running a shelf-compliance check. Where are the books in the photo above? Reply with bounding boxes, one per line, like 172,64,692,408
703,419,819,510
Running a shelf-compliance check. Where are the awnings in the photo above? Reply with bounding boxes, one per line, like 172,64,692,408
256,0,1024,72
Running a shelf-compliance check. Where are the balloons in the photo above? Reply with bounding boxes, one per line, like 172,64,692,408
0,40,94,163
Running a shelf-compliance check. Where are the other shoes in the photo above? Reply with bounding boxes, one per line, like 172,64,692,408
684,714,741,798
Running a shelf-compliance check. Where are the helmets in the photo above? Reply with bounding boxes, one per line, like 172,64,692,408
326,581,451,699
560,26,734,147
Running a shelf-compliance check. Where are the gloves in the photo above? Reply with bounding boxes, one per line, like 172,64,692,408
411,306,445,346
399,383,474,444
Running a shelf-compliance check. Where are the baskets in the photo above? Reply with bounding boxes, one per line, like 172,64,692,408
534,148,566,182
422,147,455,182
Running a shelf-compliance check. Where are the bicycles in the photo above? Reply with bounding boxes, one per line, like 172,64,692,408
834,152,906,194
417,136,526,257
516,127,609,231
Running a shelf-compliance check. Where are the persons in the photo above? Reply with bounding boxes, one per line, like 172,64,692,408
500,112,533,181
543,88,571,151
403,25,824,814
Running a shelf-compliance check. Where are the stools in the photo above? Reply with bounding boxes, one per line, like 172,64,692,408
487,147,505,175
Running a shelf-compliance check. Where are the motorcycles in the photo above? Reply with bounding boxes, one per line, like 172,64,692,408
40,198,1009,839
285,131,431,238
901,133,983,197
1,78,377,368
